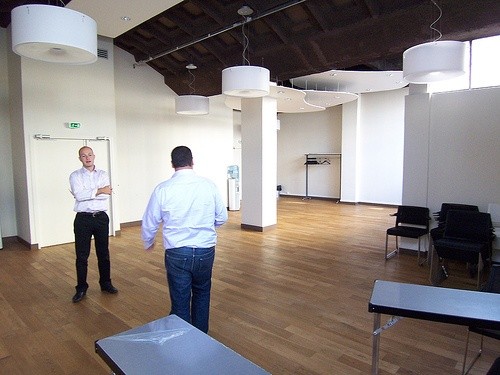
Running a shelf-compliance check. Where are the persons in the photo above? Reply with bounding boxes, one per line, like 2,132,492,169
141,146,228,334
69,146,118,302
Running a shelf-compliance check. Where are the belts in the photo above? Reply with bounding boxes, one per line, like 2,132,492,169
77,211,105,217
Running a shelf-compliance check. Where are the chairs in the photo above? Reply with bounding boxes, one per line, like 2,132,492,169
385,200,500,375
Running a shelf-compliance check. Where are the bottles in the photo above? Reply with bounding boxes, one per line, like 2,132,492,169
229,165,239,179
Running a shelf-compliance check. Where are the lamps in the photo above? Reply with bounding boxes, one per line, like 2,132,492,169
12,5,97,65
402,1,466,83
175,62,209,114
223,7,271,98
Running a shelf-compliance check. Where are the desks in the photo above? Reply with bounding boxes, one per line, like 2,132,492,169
95,313,275,375
368,279,500,375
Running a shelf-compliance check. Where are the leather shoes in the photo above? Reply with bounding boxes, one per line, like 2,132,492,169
101,283,118,293
72,290,86,302
